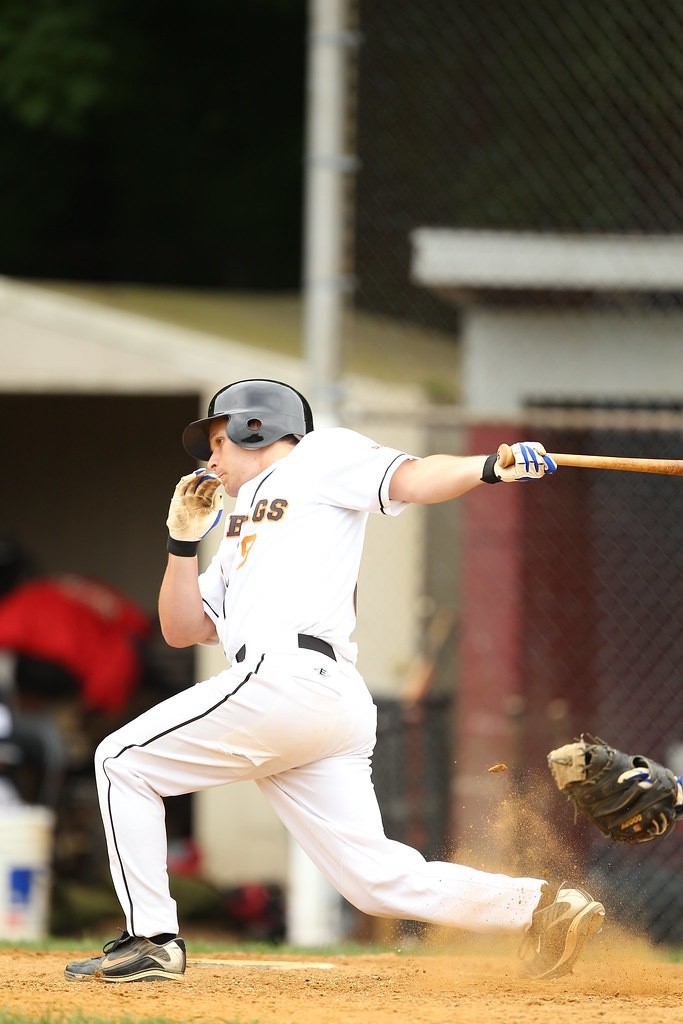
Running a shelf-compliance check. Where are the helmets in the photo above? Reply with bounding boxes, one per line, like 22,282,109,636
182,378,314,462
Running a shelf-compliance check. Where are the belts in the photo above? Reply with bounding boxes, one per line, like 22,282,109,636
234,633,338,665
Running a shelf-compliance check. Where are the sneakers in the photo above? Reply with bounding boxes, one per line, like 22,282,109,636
63,925,187,984
517,877,605,983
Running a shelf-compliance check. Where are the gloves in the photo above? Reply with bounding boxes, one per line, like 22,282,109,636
480,440,558,484
166,468,223,558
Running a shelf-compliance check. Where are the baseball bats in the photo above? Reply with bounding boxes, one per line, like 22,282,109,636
495,443,683,475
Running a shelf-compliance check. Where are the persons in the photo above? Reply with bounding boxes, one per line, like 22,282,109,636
547,742,683,846
62,379,606,983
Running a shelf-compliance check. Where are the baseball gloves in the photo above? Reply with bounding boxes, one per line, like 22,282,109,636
547,731,677,847
